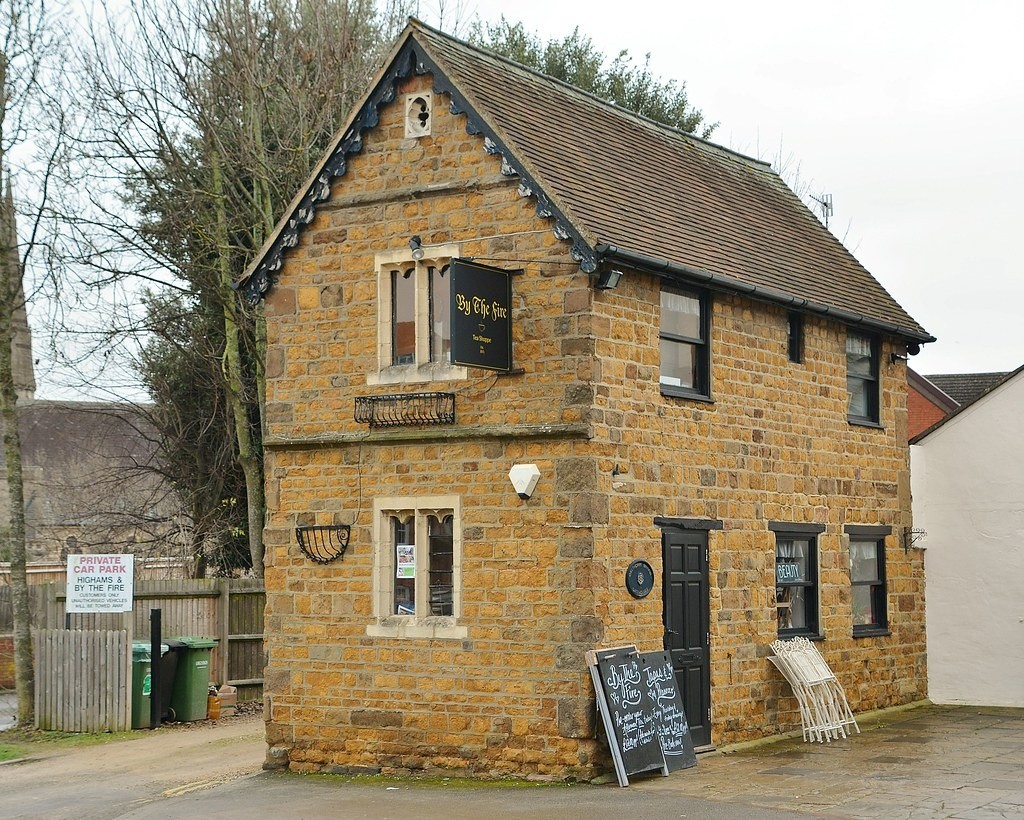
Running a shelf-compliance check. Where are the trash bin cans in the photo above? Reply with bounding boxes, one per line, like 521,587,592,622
151,640,189,729
168,636,219,723
132,640,170,730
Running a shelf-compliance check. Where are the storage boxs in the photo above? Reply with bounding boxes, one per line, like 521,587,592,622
208,685,238,719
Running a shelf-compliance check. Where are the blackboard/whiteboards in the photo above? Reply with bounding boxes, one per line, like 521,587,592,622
585,646,665,776
639,650,698,773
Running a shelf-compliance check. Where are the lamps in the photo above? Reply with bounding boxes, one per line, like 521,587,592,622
408,236,424,259
891,353,910,364
594,270,624,290
